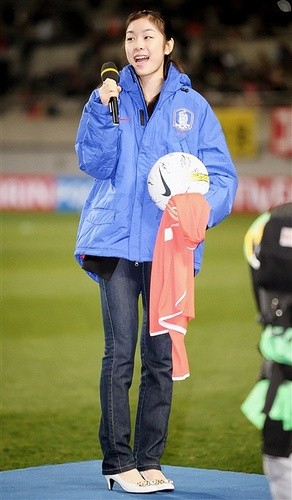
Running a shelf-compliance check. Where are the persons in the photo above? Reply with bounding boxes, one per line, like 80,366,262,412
73,10,238,494
240,203,292,500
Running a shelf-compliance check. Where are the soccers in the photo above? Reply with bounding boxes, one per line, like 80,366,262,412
147,152,210,211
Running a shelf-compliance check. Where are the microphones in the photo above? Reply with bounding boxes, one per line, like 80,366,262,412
101,62,119,124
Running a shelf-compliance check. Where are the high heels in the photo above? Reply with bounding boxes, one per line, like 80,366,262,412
105,471,158,493
140,470,174,491
